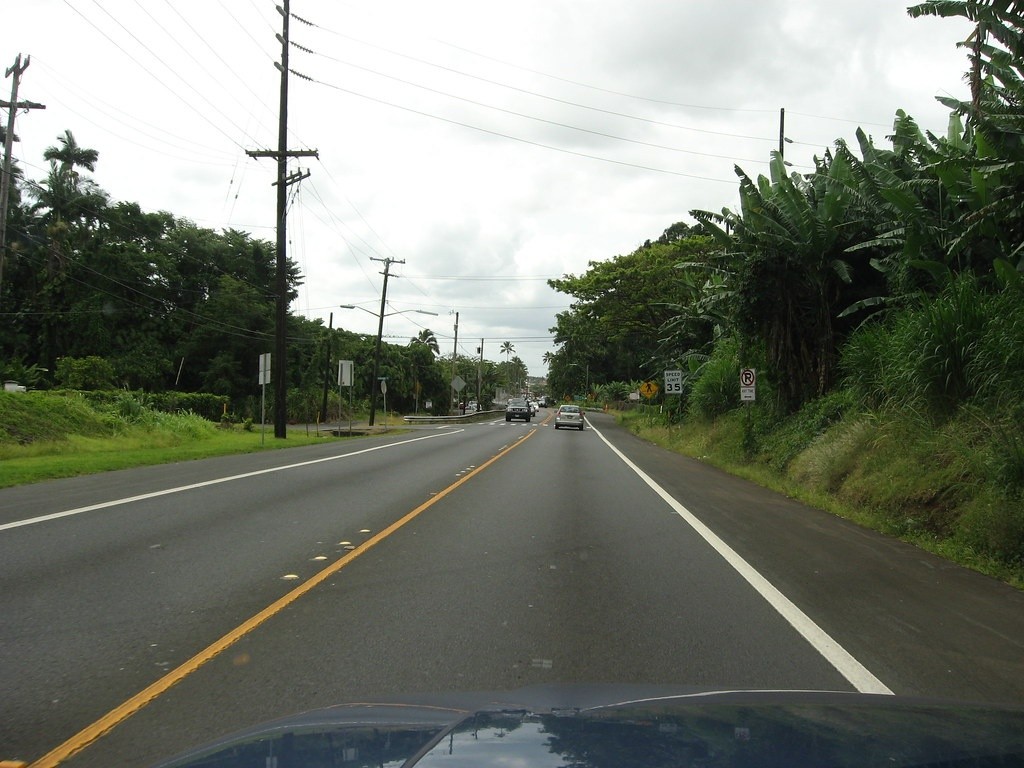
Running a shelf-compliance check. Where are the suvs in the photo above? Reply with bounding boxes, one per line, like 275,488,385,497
505,398,531,423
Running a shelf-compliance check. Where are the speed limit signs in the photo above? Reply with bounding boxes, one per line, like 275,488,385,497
664,369,683,393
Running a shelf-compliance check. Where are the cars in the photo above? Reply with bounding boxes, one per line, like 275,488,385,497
505,394,558,417
553,404,585,430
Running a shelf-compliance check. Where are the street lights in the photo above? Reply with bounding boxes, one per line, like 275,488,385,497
569,363,589,411
339,303,439,427
